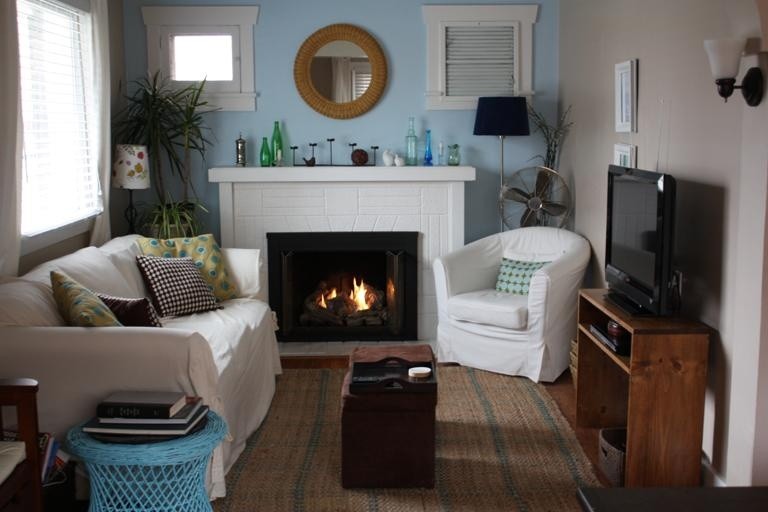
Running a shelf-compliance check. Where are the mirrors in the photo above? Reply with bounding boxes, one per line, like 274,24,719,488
293,23,386,120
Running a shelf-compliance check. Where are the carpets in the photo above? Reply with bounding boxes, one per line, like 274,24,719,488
206,364,601,512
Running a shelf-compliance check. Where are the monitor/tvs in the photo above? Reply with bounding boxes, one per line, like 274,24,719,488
604,165,677,317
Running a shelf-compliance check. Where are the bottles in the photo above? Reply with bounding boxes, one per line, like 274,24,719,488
405,116,418,166
447,144,460,166
272,121,283,166
260,137,270,167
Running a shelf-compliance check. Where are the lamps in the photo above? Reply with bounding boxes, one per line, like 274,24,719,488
703,37,763,106
111,144,152,230
472,97,530,233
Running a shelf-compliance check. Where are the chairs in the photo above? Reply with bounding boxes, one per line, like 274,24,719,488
0,377,41,512
433,226,591,384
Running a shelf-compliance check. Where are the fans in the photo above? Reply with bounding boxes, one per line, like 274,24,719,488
499,165,572,230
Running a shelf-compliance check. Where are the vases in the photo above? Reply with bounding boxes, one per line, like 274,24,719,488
447,143,459,166
424,128,433,166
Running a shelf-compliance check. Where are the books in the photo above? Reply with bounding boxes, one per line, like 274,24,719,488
80,391,209,435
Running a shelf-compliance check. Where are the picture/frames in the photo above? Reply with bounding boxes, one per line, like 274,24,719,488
612,143,637,169
613,57,640,134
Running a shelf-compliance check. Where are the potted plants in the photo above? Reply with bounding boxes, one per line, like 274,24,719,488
110,70,211,237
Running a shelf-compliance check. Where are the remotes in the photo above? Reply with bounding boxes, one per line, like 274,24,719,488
351,374,379,383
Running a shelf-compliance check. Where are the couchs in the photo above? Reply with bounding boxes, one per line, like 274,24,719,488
0,231,283,503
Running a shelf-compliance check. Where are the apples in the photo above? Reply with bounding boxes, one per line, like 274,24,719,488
350,148,369,166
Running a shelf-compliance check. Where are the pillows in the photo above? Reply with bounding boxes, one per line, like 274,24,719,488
495,256,551,295
136,233,238,303
97,293,162,327
50,269,125,327
136,256,224,316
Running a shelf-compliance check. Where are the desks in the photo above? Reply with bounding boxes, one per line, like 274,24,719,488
341,345,438,488
577,485,766,511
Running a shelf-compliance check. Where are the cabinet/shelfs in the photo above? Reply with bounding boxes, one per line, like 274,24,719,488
575,290,710,488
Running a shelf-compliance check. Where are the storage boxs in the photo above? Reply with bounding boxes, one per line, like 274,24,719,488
597,427,626,484
